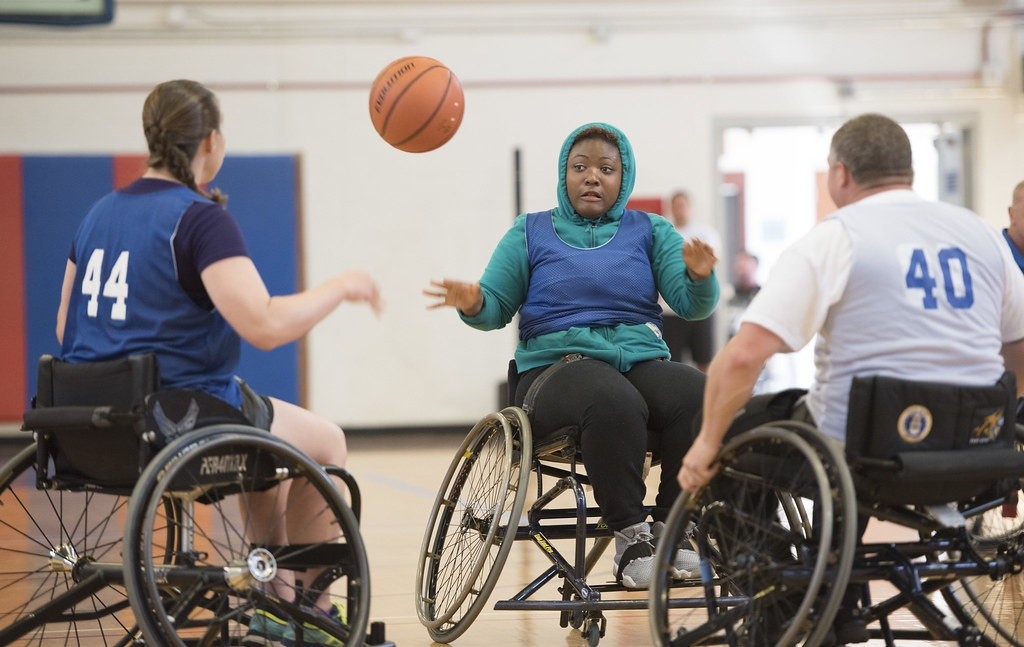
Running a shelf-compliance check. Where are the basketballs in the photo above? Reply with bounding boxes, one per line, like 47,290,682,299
368,55,465,153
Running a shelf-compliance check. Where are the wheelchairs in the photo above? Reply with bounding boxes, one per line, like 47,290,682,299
643,367,1024,647
411,359,728,646
0,346,388,646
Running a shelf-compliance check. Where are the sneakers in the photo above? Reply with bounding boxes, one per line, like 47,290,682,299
614,522,656,588
651,520,716,579
240,599,350,647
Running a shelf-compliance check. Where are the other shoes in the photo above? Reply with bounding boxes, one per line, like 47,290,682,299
734,590,868,647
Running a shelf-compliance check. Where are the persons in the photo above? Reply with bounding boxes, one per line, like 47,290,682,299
677,113,1024,647
57,80,380,647
423,124,720,588
